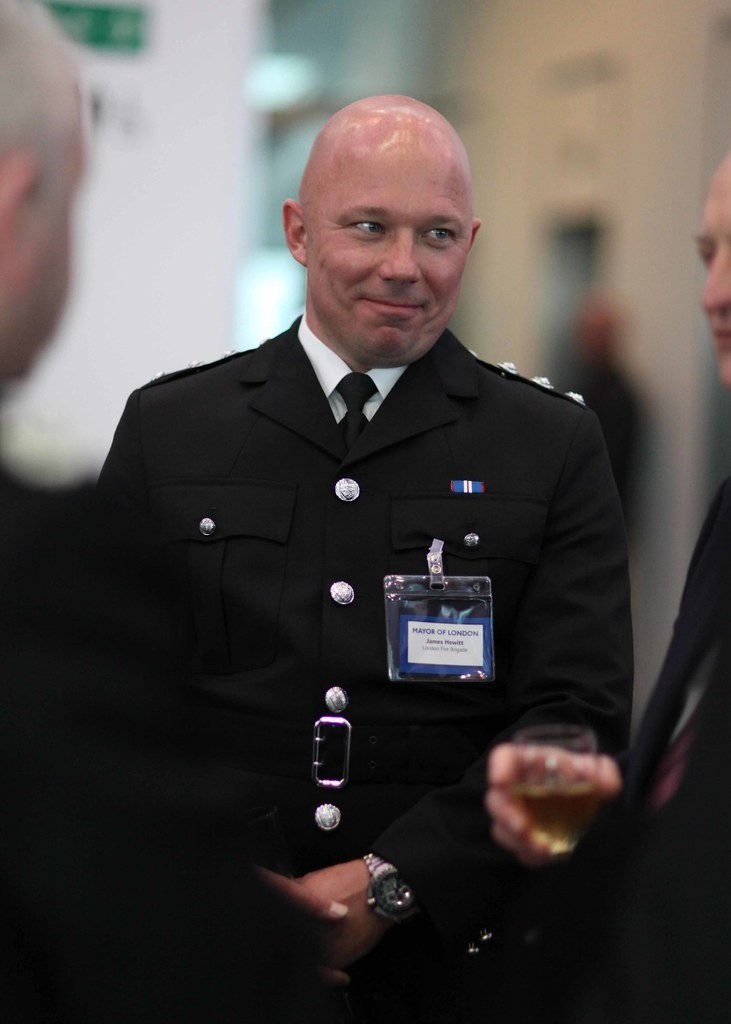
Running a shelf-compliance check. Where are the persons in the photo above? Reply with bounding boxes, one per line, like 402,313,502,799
2,0,113,1024
536,208,652,532
484,151,728,1024
90,92,636,1023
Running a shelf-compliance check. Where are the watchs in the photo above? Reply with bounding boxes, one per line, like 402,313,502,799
363,849,417,926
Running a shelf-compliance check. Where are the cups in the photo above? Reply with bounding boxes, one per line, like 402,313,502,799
513,725,601,857
